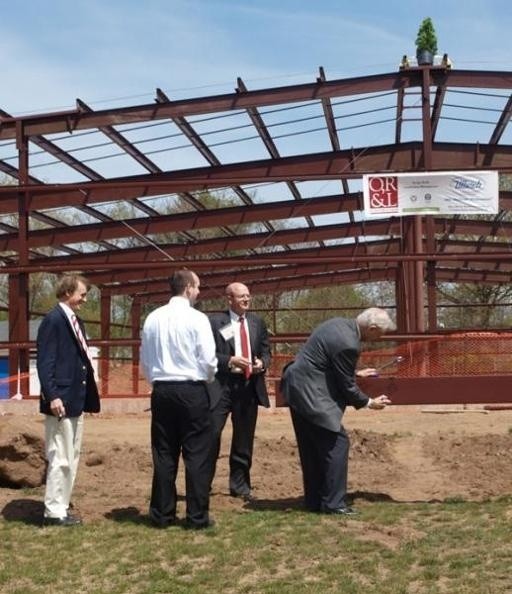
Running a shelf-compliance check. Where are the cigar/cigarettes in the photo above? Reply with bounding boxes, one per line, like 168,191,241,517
59,414,62,418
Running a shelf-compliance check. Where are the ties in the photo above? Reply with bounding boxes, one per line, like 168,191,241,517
238,317,251,379
71,314,92,364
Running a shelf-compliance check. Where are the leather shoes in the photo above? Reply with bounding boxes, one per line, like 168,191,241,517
43,514,82,527
327,505,362,516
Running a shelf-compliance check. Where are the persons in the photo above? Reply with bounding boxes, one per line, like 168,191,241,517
139,268,218,530
36,275,100,527
207,281,272,499
280,307,391,516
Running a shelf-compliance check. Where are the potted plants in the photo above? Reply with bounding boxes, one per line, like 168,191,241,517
415,17,439,65
